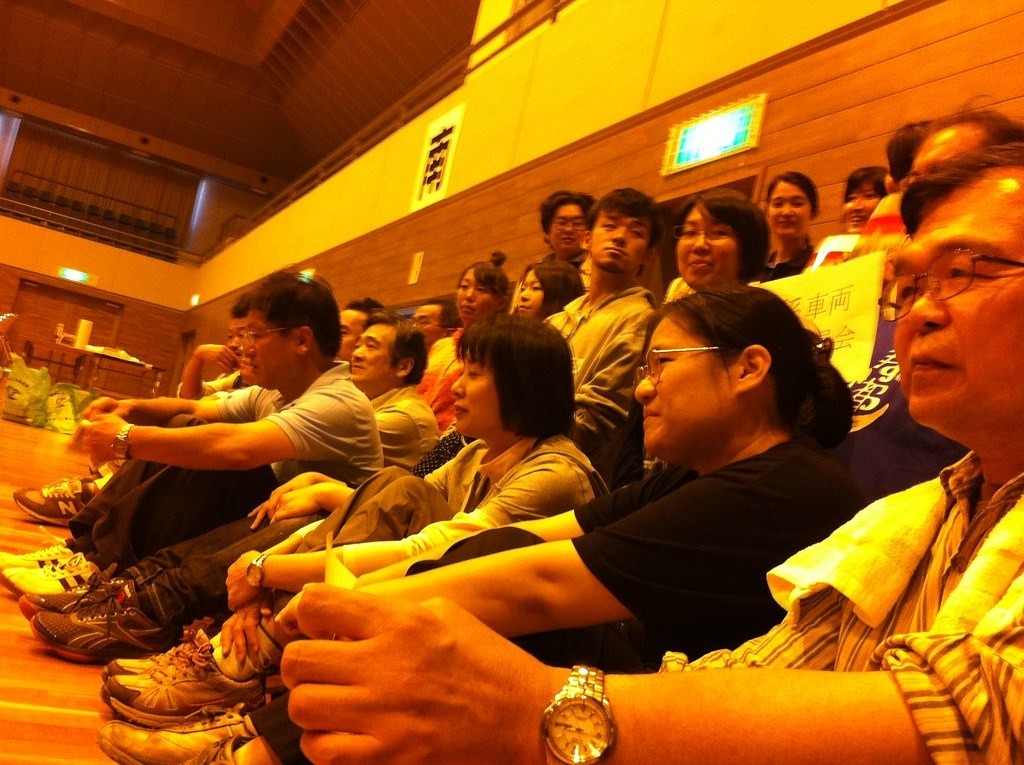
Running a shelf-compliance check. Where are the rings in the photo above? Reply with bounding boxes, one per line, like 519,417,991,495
224,359,228,364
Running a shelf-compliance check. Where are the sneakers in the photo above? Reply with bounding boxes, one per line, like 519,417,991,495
3,552,101,597
18,564,128,621
101,631,265,729
96,703,255,765
13,477,100,526
30,579,177,663
1,526,75,573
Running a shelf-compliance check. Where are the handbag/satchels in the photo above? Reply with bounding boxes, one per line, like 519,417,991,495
2,352,105,434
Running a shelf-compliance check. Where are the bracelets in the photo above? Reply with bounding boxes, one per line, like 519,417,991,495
110,423,135,459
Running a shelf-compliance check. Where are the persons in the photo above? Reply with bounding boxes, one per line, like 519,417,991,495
1,96,1024,765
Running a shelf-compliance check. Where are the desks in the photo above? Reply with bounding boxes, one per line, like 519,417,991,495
52,342,167,398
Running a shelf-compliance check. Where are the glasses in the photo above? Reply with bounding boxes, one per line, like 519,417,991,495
672,224,738,239
878,247,1024,322
237,323,316,345
410,317,450,328
234,336,247,351
551,218,589,230
904,171,922,190
634,347,739,385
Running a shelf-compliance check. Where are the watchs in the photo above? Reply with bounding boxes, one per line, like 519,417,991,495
246,553,270,588
540,664,617,765
